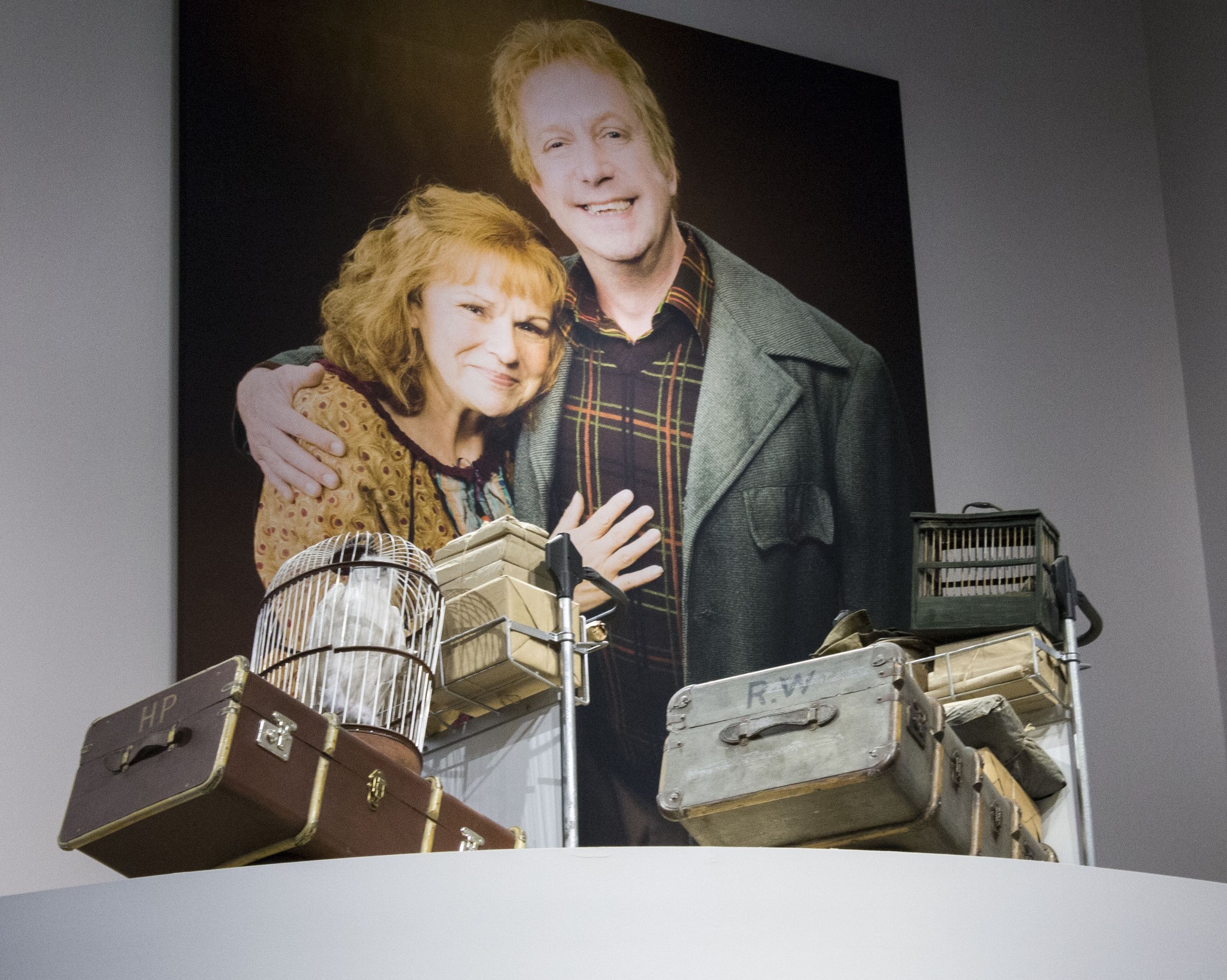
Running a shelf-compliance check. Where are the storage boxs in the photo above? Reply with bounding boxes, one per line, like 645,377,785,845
970,747,1046,847
931,627,1071,712
906,502,1072,636
55,656,527,882
424,574,590,720
656,641,1061,867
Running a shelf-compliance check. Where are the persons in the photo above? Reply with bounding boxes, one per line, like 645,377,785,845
229,20,916,848
253,185,663,851
